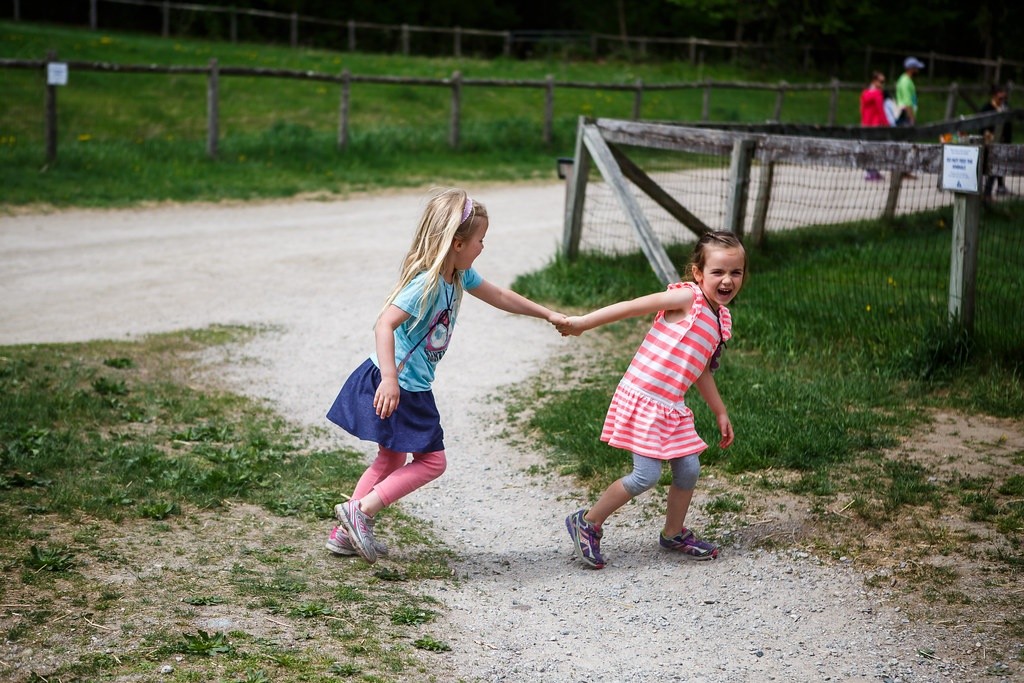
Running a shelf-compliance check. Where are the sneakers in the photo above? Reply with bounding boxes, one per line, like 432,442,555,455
565,509,606,570
659,527,718,560
326,526,390,559
333,497,377,564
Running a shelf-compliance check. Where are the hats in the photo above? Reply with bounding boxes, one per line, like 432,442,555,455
904,58,924,69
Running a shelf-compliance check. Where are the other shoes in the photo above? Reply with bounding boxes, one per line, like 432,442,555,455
903,173,919,179
996,189,1012,196
866,172,885,179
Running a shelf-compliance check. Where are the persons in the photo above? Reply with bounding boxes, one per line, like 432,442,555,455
897,57,925,179
979,83,1012,195
325,188,569,562
859,72,889,179
557,230,746,568
